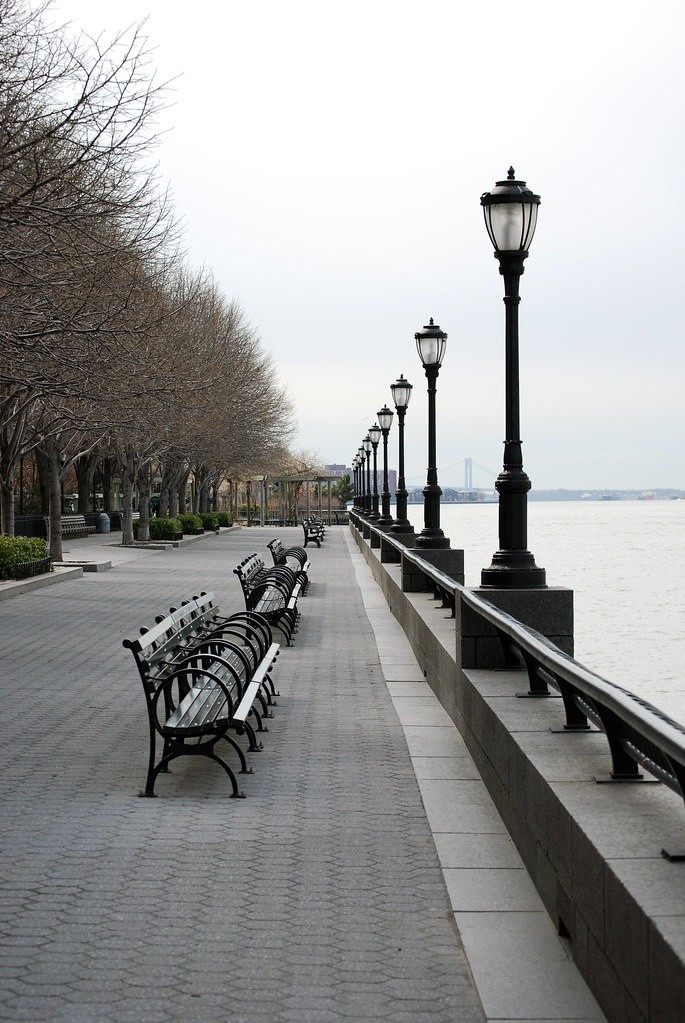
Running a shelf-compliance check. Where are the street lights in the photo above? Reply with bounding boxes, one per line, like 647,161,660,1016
358,444,367,513
478,165,546,590
367,422,381,520
413,317,454,550
351,452,362,512
376,403,395,525
362,434,373,517
389,374,414,534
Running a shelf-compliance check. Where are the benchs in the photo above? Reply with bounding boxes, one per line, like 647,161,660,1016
233,553,301,648
44,515,96,542
120,512,140,530
123,591,280,798
267,538,311,597
301,514,325,548
